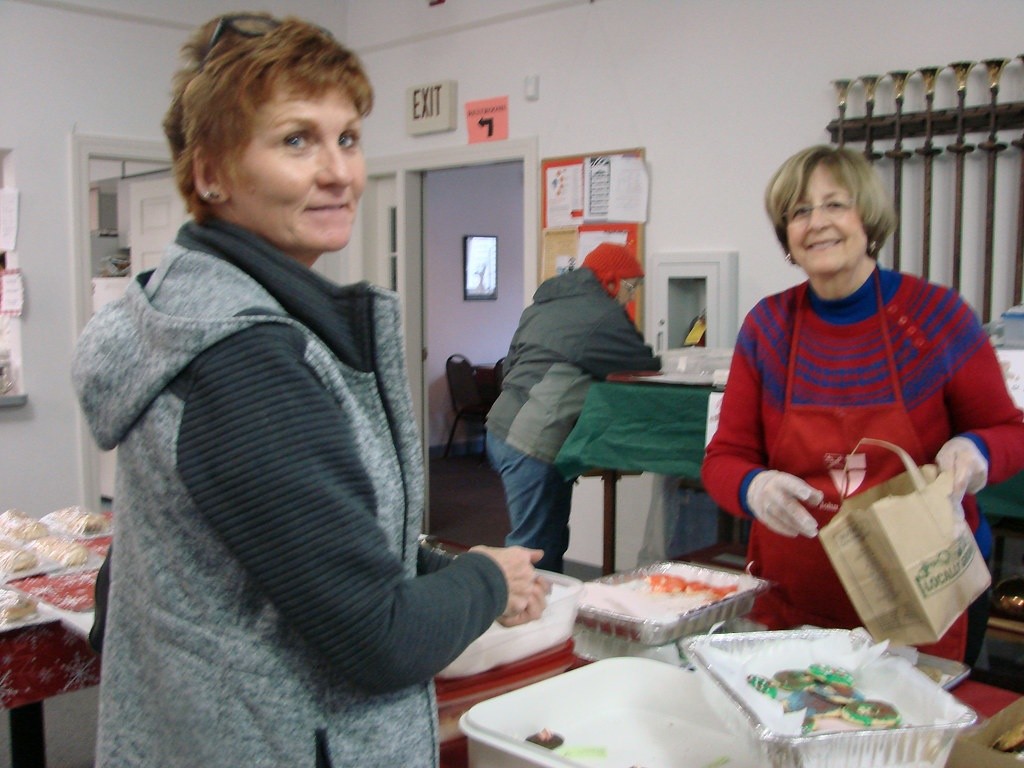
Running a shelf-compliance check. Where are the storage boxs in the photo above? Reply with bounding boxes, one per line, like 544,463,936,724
441,557,980,768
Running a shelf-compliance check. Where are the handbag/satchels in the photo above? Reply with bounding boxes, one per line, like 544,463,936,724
816,436,992,644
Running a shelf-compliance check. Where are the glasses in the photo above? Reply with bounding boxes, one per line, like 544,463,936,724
784,197,857,229
622,279,637,295
209,13,333,52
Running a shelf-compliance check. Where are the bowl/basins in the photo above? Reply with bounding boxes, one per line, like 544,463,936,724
439,557,980,768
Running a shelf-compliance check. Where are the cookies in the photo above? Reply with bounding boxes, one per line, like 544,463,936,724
748,661,900,734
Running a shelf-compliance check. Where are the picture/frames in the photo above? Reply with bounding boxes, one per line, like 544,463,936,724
462,235,500,302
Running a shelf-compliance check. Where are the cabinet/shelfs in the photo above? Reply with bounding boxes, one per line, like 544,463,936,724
560,252,738,569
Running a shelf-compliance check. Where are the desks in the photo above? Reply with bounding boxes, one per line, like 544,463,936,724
555,376,739,573
0,507,112,768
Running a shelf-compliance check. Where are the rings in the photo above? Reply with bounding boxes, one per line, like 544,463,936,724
762,500,775,514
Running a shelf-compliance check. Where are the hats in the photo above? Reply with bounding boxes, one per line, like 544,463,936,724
580,242,645,298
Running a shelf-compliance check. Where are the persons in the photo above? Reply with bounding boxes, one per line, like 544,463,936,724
72,17,553,768
700,144,1024,668
485,242,662,574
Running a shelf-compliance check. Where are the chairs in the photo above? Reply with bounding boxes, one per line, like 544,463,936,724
440,353,482,465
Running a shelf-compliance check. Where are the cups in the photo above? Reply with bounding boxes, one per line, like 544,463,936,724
1002,304,1024,351
